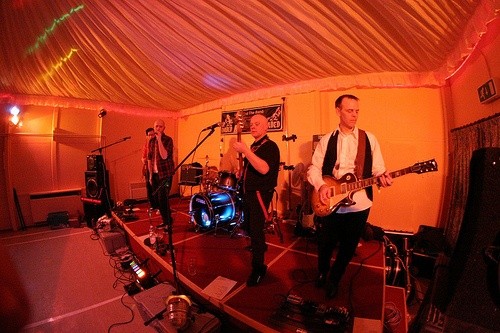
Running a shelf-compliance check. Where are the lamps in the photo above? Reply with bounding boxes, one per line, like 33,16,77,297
167,295,196,331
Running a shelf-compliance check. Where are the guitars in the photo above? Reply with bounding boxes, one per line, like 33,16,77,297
143,158,148,176
235,131,244,211
310,159,439,217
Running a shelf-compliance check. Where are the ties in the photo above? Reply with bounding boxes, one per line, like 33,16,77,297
156,140,158,166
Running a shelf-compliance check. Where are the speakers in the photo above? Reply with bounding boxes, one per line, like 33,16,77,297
86,155,103,171
179,164,202,184
84,171,110,200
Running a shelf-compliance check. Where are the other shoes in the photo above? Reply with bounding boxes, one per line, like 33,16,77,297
317,267,330,286
156,209,160,215
163,229,168,235
155,223,168,229
326,277,339,298
247,268,264,287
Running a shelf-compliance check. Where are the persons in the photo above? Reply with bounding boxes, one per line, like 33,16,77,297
232,114,280,287
306,94,393,298
144,119,175,234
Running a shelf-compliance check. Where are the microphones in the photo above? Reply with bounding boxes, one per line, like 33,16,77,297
120,136,131,141
203,122,222,131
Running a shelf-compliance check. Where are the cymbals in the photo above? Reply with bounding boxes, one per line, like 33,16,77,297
189,166,219,173
194,172,209,178
198,178,216,180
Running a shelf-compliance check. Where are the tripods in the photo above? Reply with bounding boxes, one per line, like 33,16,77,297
141,130,208,328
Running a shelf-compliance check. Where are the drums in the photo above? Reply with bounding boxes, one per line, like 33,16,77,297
189,191,235,229
218,171,236,190
